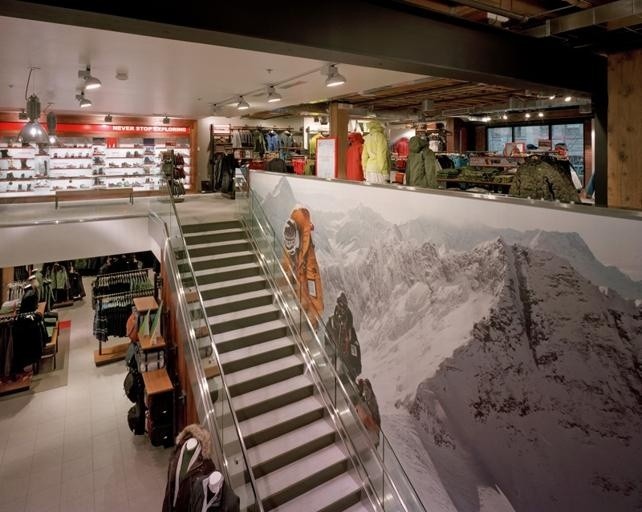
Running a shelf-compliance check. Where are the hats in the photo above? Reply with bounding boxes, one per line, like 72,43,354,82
284,219,297,244
337,292,348,308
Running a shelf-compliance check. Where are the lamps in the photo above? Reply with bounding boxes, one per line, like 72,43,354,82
19,110,27,119
42,104,62,147
78,67,100,89
105,113,112,122
16,66,50,144
76,92,91,107
216,62,345,114
163,116,169,124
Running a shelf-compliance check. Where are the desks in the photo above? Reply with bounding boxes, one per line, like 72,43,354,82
143,369,174,450
138,333,165,371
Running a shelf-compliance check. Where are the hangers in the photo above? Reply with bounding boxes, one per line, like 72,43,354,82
93,270,154,309
1,312,34,320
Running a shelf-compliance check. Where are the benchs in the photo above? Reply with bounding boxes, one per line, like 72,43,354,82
55,188,134,209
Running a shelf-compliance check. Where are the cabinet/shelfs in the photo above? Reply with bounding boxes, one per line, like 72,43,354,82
212,125,304,199
0,107,197,203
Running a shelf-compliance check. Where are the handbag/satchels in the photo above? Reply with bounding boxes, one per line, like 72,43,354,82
123,313,140,432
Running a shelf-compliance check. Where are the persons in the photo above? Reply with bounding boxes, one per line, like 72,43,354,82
406,136,439,189
18,267,43,312
162,438,206,512
361,124,390,184
189,471,240,512
346,132,366,181
277,205,381,449
